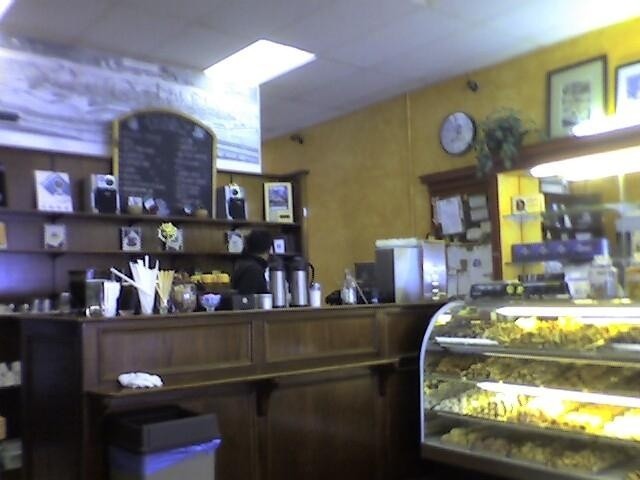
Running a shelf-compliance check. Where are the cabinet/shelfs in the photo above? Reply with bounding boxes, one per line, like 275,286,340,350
0,206,298,259
418,294,640,478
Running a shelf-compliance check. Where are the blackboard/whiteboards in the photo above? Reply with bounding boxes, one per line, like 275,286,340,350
112,106,217,220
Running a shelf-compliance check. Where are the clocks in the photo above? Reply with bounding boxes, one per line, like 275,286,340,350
437,111,476,156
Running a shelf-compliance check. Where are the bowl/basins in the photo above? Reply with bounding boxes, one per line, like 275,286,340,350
198,294,222,312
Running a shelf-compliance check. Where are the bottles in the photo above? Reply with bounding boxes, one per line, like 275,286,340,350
270,256,288,307
341,272,358,304
289,257,314,306
308,282,322,306
590,256,618,298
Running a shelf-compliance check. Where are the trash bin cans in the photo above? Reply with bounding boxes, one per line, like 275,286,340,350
103,404,224,480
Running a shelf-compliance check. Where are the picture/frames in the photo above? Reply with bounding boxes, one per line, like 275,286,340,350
545,52,640,139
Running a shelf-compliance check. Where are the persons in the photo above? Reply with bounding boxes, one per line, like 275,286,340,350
231,229,272,295
